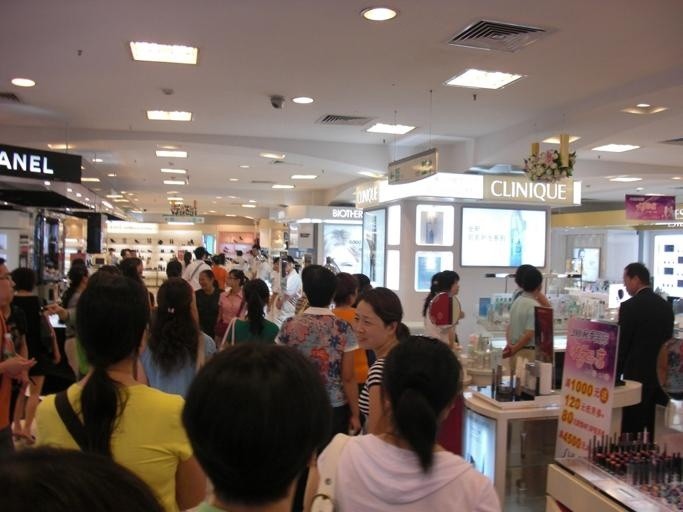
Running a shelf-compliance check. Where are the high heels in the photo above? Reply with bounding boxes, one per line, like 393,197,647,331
12,429,21,441
19,431,36,444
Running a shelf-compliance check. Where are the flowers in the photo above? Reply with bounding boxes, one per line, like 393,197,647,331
521,150,576,181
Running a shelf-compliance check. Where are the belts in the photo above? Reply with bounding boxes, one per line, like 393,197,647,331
521,346,535,350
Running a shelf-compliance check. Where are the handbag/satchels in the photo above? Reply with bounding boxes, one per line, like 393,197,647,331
219,317,236,351
38,297,56,355
308,433,350,511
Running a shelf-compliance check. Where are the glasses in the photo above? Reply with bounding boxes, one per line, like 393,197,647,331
0,273,12,281
227,276,238,281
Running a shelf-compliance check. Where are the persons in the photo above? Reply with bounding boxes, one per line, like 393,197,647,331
0,256,37,455
121,249,131,260
216,268,250,346
351,286,404,437
181,252,194,269
312,335,501,512
166,261,199,328
502,266,543,373
352,274,373,307
273,256,302,325
60,263,90,383
194,270,226,340
42,265,121,329
77,250,84,259
33,266,208,512
423,270,465,360
268,257,282,313
108,248,118,266
332,271,370,395
251,244,268,280
143,275,217,399
225,279,280,347
236,250,246,265
281,263,362,445
0,447,165,512
510,264,550,307
11,266,60,445
211,254,229,293
181,344,334,512
617,262,675,435
423,272,441,324
182,246,211,291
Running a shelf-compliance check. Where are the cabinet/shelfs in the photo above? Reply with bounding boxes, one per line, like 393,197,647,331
215,231,254,256
106,231,199,287
